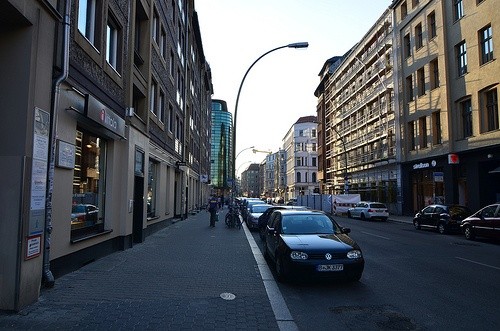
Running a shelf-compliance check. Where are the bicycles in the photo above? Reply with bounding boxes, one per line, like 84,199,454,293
225,203,245,230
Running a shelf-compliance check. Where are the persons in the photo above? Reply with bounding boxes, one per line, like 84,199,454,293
205,194,217,227
268,200,273,205
206,192,230,210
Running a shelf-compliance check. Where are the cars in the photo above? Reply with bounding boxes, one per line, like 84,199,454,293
286,198,298,206
262,209,366,283
347,201,391,222
412,203,474,235
459,202,500,243
244,200,266,221
242,197,260,208
246,204,274,233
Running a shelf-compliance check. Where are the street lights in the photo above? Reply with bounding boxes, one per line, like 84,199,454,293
312,119,350,189
229,40,309,224
